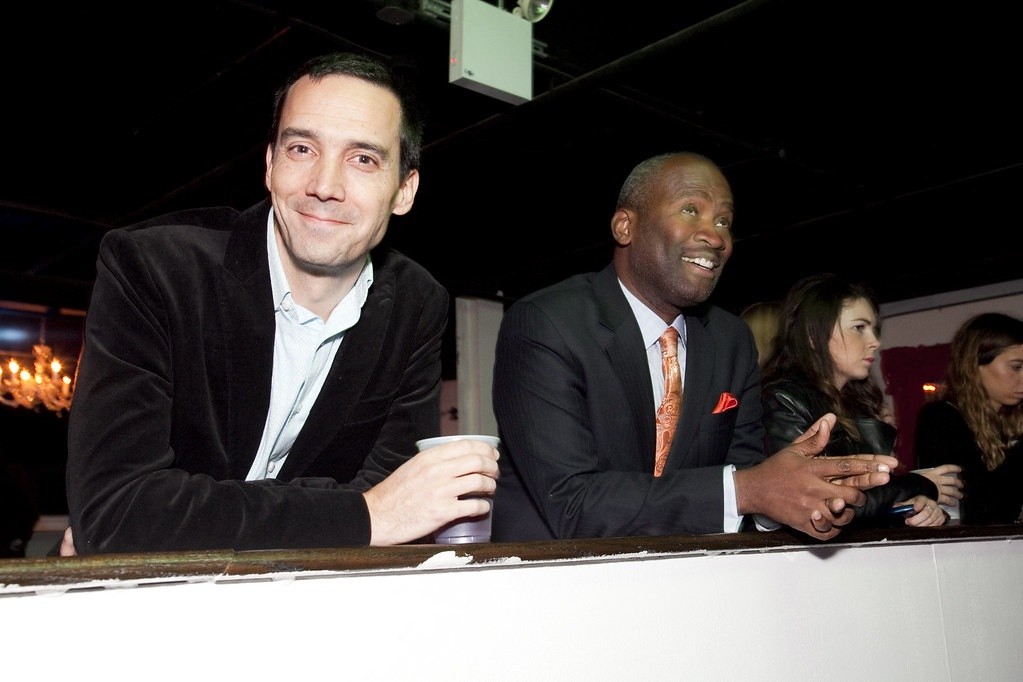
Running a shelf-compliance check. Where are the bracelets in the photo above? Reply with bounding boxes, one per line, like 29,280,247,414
941,509,951,524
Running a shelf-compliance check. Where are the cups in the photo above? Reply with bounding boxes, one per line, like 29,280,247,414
415,434,500,544
910,468,960,524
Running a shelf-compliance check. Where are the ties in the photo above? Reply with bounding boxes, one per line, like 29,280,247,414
652,326,682,478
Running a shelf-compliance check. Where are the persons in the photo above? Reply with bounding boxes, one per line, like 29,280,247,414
912,312,1023,525
758,278,964,529
61,53,501,556
490,151,899,543
